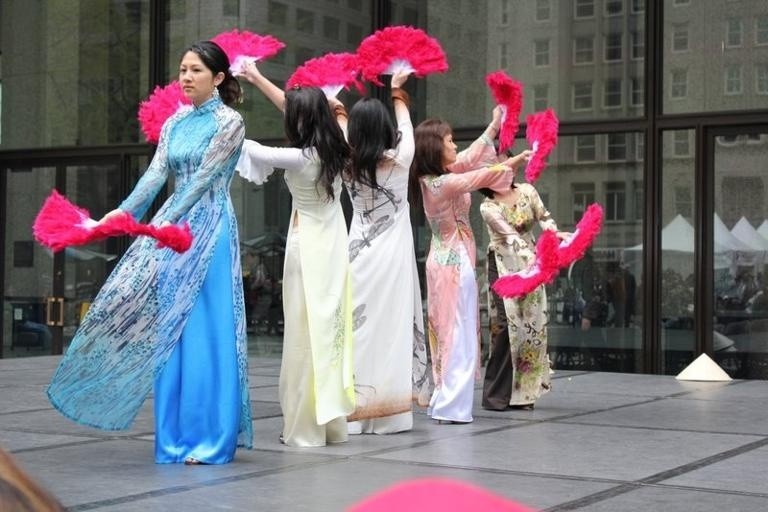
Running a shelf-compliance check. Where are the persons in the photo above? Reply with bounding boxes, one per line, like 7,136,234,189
235,58,350,448
413,105,534,424
325,70,432,435
247,251,273,358
546,261,767,325
100,41,244,466
478,146,569,412
